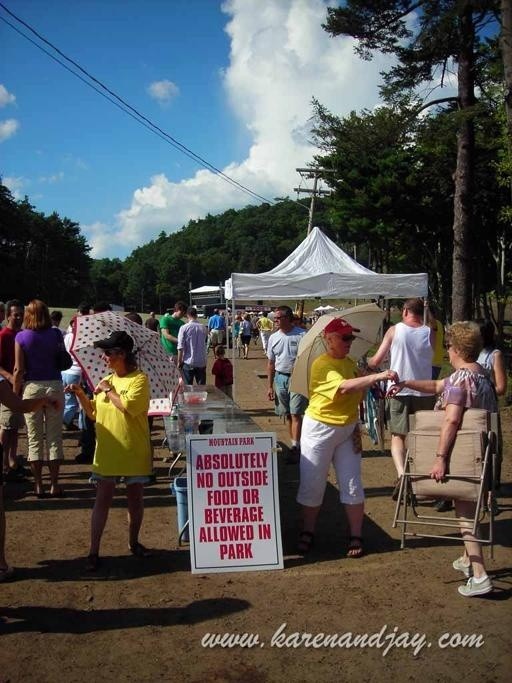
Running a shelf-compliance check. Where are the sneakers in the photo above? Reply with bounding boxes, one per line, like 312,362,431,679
458,576,494,596
1,567,15,578
391,477,402,499
128,544,145,554
452,556,473,576
76,454,93,463
434,497,453,512
284,446,300,464
85,554,99,571
3,464,31,483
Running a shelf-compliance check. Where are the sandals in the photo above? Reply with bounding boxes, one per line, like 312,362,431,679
347,537,363,557
296,532,314,552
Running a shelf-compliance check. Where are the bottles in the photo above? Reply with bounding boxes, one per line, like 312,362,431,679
170,406,179,434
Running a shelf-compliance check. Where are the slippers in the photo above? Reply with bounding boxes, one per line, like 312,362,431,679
34,487,63,498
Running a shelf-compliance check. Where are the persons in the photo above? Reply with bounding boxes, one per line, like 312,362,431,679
62,331,152,573
11,299,73,498
432,320,507,519
0,375,65,581
385,320,498,597
1,300,447,483
364,298,439,502
293,319,400,559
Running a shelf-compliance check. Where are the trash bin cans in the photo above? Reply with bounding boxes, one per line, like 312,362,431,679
170,477,187,542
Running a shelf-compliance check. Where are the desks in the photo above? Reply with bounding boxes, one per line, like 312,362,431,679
162,385,282,546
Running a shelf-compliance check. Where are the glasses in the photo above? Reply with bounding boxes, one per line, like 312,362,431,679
445,343,453,350
339,334,357,342
273,316,287,320
105,351,113,356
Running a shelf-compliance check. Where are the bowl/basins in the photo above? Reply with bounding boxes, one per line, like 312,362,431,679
184,392,208,403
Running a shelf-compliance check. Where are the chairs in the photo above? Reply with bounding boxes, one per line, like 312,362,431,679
392,409,501,559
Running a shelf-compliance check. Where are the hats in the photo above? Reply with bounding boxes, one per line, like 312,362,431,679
95,331,134,352
325,319,360,337
